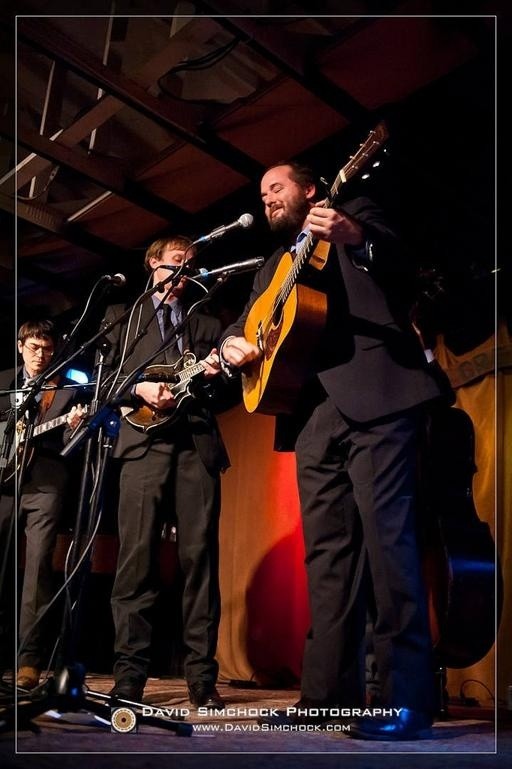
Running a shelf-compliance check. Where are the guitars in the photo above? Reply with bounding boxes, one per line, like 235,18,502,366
243,121,389,412
119,349,221,435
0,402,92,483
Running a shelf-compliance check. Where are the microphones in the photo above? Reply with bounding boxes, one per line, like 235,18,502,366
105,272,127,287
197,255,265,281
199,213,254,240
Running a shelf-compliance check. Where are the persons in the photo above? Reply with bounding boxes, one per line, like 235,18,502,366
88,235,239,714
216,160,460,743
408,319,458,408
0,314,85,691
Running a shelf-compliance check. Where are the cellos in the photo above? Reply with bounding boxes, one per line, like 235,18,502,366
408,266,505,667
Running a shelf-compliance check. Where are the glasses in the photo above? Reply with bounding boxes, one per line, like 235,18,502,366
22,344,54,356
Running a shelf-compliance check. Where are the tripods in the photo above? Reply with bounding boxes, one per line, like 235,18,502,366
0,451,194,740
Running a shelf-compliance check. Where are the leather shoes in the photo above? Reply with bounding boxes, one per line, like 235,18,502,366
346,704,435,741
17,666,41,689
108,676,145,706
259,698,365,730
188,682,225,710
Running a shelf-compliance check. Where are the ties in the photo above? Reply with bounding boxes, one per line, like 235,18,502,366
159,304,181,366
